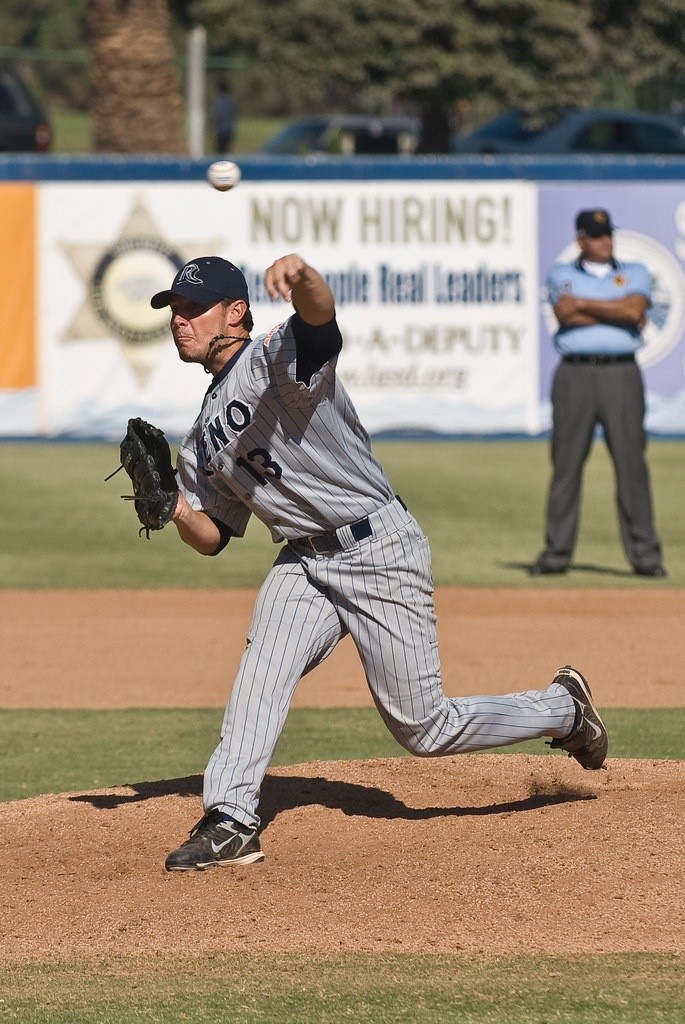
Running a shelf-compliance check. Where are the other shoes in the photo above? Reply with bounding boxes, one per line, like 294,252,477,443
636,564,664,578
530,563,561,575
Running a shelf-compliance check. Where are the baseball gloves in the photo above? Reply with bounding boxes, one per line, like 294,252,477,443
119,417,179,530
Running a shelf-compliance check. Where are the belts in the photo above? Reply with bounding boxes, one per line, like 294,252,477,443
297,495,408,556
564,352,635,366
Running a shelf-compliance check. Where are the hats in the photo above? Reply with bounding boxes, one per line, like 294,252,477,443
150,256,248,306
577,209,615,236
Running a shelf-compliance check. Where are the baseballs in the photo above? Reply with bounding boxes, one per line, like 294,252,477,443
208,160,241,191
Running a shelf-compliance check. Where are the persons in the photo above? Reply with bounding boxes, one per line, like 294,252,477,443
211,80,240,154
122,254,607,869
530,210,665,577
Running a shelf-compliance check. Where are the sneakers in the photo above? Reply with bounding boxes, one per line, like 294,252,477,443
544,666,609,770
165,808,265,872
